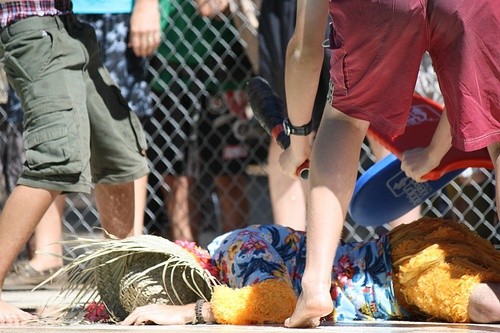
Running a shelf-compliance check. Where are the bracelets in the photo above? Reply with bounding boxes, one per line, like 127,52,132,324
194,298,208,324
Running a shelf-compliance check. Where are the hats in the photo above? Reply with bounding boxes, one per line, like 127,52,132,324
93,234,213,323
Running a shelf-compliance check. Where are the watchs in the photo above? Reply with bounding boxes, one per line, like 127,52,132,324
282,118,314,136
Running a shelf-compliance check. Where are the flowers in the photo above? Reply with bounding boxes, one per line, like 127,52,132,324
85,302,113,325
174,239,223,283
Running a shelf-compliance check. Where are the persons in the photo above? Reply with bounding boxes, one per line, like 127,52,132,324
279,0,499,329
256,0,422,237
86,214,499,325
1,1,260,295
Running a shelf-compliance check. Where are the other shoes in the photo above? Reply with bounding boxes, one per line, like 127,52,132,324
3,260,68,287
